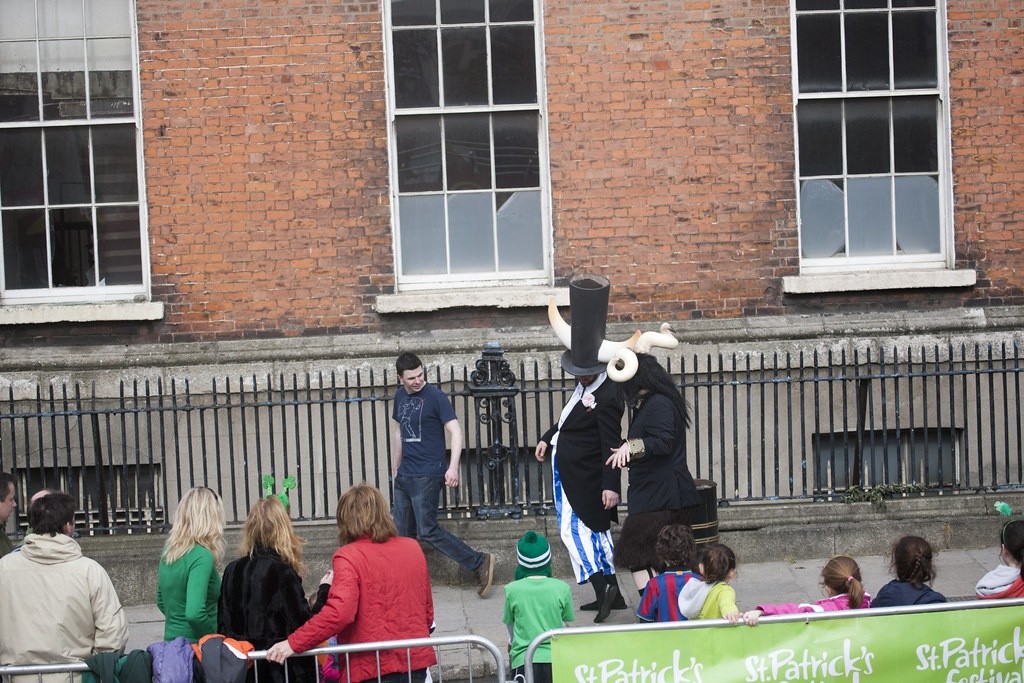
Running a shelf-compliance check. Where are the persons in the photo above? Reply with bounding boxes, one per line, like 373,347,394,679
869,536,948,607
635,523,743,626
156,486,437,683
743,555,872,627
974,520,1024,599
502,530,575,683
392,350,495,599
533,325,703,623
1,470,129,683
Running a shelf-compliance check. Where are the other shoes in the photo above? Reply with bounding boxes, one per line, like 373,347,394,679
475,553,494,597
580,598,627,610
594,584,618,623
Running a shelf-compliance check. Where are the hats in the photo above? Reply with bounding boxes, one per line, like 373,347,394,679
548,273,641,376
515,531,552,580
